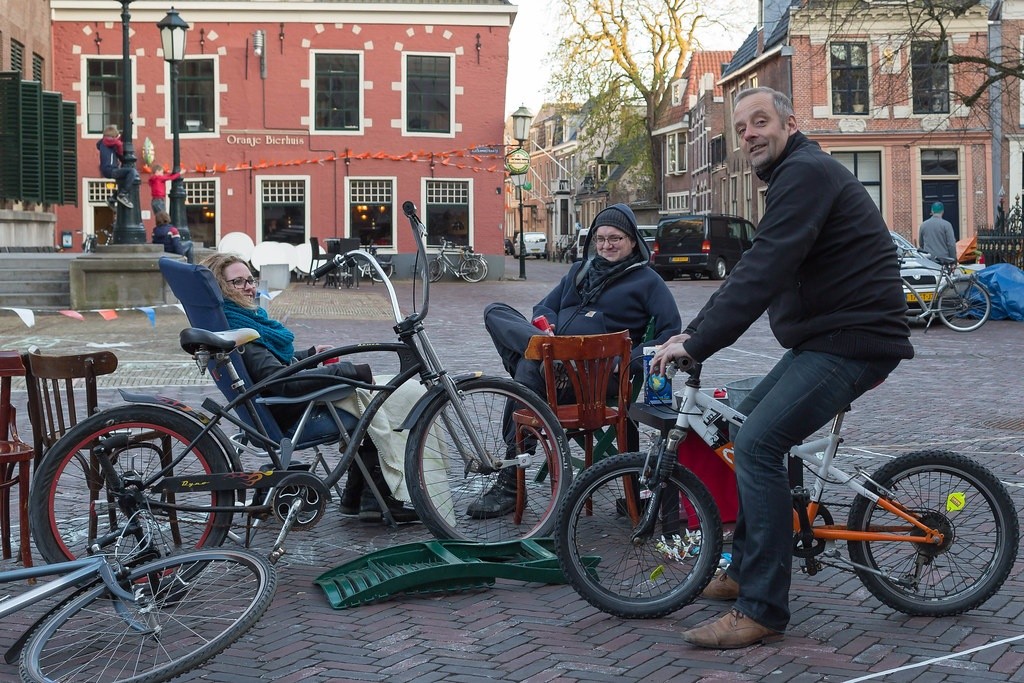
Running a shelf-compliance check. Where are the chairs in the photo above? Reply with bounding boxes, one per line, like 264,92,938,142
531,316,655,483
0,345,183,584
159,256,400,548
307,537,602,610
512,328,640,528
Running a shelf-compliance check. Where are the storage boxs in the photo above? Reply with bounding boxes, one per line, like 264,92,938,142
643,347,672,406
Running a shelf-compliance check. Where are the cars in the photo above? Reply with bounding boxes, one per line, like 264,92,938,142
554,228,590,264
889,230,987,326
504,238,513,255
637,224,658,261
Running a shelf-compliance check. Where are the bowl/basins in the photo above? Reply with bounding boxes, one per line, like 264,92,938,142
673,387,730,410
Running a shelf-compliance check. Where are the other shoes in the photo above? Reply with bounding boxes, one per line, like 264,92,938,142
117,195,134,209
106,199,117,213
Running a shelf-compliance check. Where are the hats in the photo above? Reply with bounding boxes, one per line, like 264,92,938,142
592,209,636,241
930,200,944,213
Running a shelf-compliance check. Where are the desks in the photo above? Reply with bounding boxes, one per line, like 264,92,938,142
626,403,729,538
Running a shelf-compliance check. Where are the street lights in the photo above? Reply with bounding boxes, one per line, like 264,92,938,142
154,6,193,242
509,170,527,280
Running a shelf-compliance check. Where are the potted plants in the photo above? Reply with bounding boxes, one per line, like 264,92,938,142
851,71,866,113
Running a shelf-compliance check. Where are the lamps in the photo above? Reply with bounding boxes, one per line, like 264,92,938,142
280,23,284,54
430,152,435,179
476,33,481,63
344,149,349,175
200,28,203,53
94,31,101,54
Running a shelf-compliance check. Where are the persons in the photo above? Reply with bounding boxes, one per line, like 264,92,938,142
466,203,682,518
647,88,915,648
917,201,957,266
197,254,456,529
99,126,136,212
148,166,194,263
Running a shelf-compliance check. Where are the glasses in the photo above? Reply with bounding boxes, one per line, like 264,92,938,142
592,235,628,245
225,276,259,289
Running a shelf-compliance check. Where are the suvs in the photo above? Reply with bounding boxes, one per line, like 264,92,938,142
513,232,548,259
653,213,757,281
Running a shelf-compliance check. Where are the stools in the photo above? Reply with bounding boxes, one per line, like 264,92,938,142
308,236,374,289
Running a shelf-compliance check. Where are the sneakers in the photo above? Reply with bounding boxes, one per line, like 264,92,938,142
701,571,740,601
682,607,785,649
338,490,359,517
358,493,420,523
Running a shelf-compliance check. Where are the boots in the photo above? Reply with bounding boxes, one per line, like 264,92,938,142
465,462,528,519
539,358,588,388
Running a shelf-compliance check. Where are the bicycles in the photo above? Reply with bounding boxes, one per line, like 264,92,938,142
27,198,574,600
0,433,280,683
421,237,490,283
343,247,394,283
552,352,1021,619
76,228,113,254
891,235,992,334
